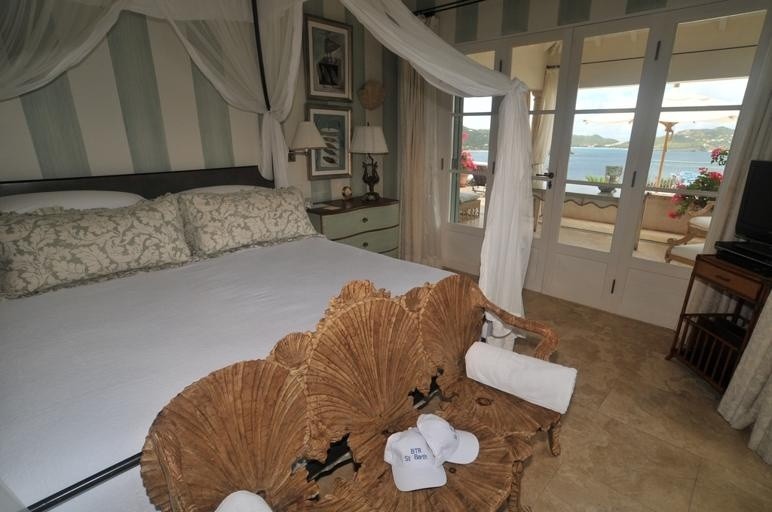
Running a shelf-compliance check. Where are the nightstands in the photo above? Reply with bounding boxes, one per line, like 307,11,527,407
306,197,400,259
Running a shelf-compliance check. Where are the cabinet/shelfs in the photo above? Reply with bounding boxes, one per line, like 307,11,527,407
665,253,772,396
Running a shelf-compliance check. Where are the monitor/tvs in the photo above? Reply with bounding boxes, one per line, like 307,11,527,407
736,160,772,245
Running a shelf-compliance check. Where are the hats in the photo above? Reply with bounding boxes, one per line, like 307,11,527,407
416,412,481,467
383,425,449,492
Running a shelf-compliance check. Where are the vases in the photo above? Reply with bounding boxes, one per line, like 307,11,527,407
460,173,467,187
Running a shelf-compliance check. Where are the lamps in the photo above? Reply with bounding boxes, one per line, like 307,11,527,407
288,121,328,162
347,124,389,201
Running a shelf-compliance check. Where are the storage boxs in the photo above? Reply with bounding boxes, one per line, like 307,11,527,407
460,200,480,223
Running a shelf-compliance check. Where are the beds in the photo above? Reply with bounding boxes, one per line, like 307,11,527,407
0,165,460,512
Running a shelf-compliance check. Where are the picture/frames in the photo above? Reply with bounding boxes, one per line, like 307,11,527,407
302,13,354,103
304,103,353,181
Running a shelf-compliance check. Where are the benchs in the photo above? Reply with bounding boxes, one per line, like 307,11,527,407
664,201,716,266
139,273,561,512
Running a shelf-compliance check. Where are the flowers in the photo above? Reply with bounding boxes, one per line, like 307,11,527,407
460,131,478,173
668,148,730,219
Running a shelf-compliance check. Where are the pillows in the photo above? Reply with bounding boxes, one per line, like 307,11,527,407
464,341,578,416
214,489,272,512
0,190,146,213
174,186,318,259
0,192,192,300
179,185,272,193
460,190,480,204
